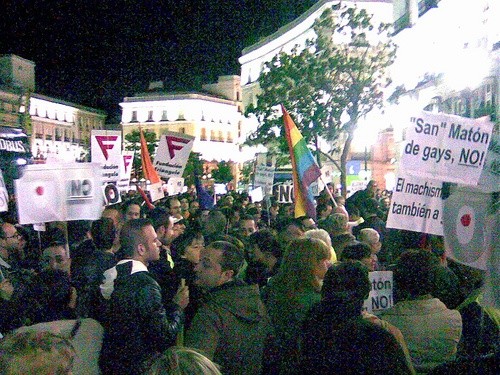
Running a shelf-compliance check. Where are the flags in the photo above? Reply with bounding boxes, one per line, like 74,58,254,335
282,104,322,221
138,126,161,185
193,166,215,209
135,183,153,210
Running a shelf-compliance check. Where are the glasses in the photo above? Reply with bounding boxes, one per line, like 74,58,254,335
3,232,20,239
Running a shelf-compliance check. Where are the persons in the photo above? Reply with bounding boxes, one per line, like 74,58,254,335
1,181,500,374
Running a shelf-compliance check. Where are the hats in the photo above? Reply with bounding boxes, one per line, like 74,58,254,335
172,218,182,224
358,228,379,244
388,249,437,274
